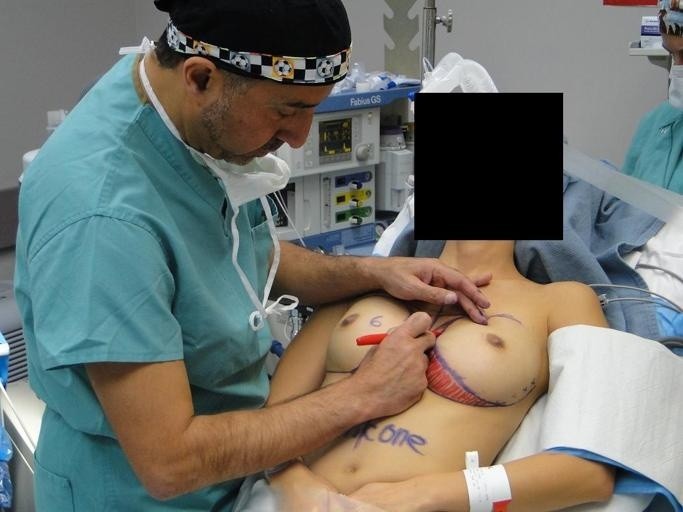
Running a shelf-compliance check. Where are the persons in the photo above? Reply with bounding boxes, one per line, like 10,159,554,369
619,0,683,198
236,240,615,512
13,1,494,512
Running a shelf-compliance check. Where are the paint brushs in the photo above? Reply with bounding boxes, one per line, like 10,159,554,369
357,326,444,345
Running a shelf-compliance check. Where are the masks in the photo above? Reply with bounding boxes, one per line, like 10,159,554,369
190,147,293,207
669,54,683,108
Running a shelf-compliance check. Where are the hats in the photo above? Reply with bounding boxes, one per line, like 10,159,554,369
657,0,682,36
153,1,351,86
